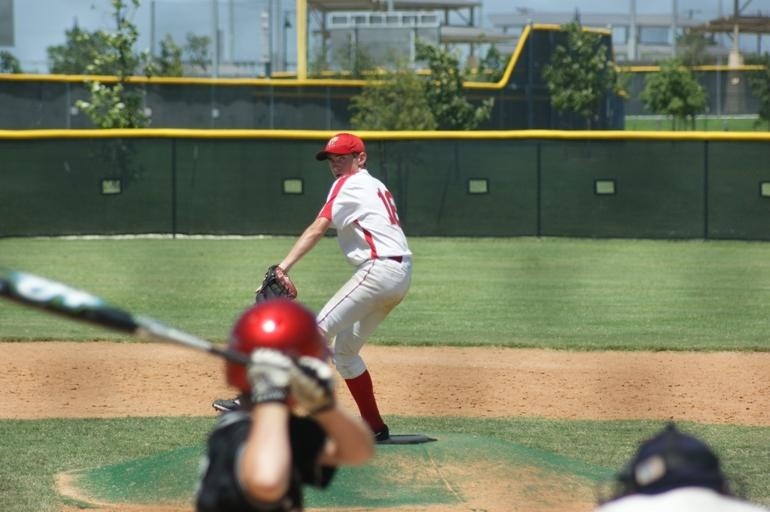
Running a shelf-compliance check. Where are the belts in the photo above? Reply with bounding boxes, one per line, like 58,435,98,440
388,255,403,263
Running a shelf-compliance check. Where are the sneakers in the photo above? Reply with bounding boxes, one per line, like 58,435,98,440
213,398,243,414
369,424,391,441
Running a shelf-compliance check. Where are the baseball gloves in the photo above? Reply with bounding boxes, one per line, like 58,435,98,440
255,265,297,304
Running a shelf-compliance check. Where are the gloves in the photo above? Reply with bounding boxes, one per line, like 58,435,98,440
290,355,335,415
243,345,295,404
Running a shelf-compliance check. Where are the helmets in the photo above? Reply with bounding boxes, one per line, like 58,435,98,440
224,298,333,389
617,422,727,492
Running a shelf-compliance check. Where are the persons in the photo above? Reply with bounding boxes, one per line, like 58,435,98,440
192,297,380,512
592,419,769,512
254,132,417,448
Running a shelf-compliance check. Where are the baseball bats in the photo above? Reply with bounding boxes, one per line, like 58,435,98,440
1,267,250,367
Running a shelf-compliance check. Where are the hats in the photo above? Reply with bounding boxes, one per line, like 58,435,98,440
315,133,366,162
